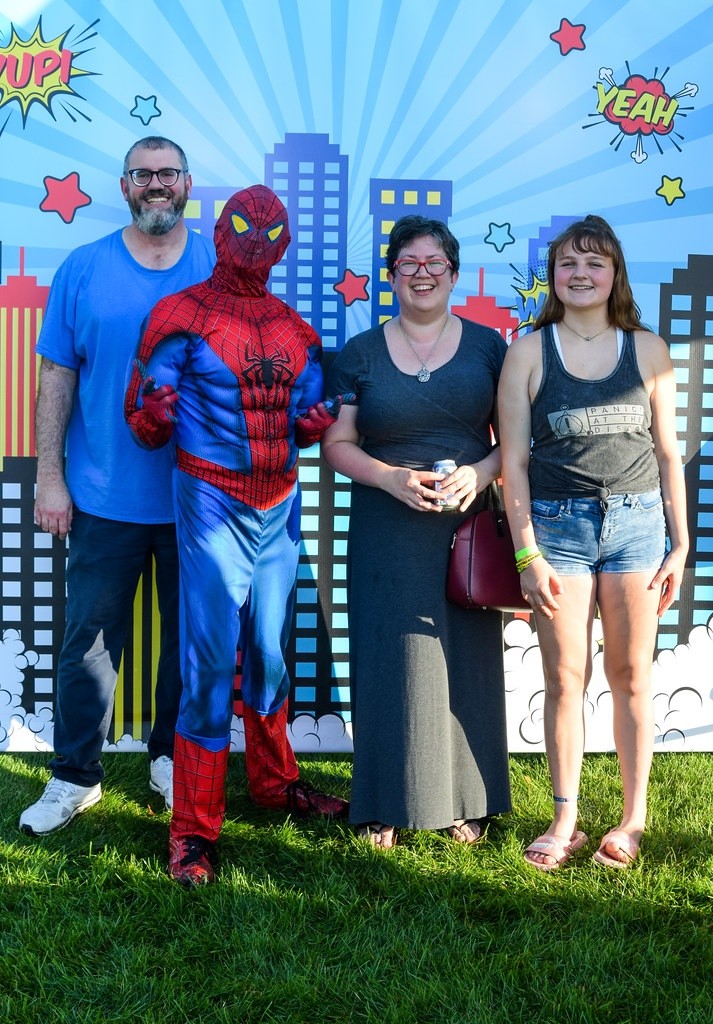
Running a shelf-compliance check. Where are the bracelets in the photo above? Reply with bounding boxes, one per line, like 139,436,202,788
515,545,538,562
517,552,541,573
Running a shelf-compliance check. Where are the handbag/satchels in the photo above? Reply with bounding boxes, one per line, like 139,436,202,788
450,484,535,610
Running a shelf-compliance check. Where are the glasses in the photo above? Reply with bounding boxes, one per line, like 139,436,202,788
122,167,181,187
391,258,453,277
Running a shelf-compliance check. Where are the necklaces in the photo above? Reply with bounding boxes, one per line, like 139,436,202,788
562,319,612,341
399,311,448,382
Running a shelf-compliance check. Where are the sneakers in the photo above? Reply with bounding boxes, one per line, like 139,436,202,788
17,777,103,836
168,828,219,885
248,778,349,817
147,754,174,808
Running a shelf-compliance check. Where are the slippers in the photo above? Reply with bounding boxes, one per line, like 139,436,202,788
356,822,398,850
446,818,482,846
523,828,589,869
593,824,643,870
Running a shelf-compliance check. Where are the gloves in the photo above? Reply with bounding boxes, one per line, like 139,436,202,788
133,361,179,424
293,392,356,435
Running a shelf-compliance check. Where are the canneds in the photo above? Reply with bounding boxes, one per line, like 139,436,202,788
431,459,461,512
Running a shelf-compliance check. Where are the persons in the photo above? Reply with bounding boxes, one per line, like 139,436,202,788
123,184,342,887
316,215,510,855
498,216,689,872
17,137,220,840
